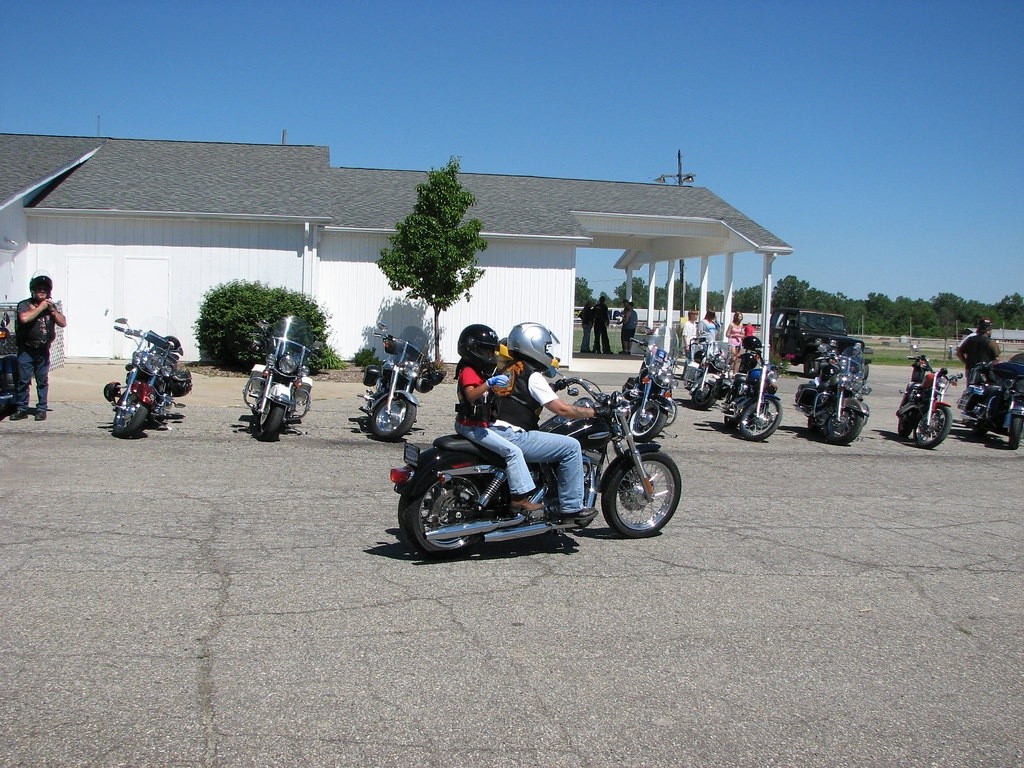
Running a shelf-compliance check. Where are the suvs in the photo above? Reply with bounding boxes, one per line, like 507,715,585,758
771,307,864,379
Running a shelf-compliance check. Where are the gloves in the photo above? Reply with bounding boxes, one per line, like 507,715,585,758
486,375,509,389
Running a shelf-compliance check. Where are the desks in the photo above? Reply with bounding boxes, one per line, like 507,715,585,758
631,333,674,356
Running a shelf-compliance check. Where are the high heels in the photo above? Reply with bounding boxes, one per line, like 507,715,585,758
511,497,543,513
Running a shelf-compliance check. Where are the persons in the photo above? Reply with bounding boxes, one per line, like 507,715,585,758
698,309,719,345
726,311,745,376
488,321,612,525
956,316,1002,387
682,310,699,378
455,323,544,511
618,298,637,354
593,296,614,354
579,299,593,353
10,275,67,421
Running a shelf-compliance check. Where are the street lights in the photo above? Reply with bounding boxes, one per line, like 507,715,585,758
655,149,697,319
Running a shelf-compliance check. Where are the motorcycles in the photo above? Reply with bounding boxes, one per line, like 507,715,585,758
896,354,964,449
726,346,790,442
622,324,680,444
112,317,187,438
357,320,435,440
964,360,1024,451
684,334,734,410
389,356,680,560
242,314,322,441
794,343,871,444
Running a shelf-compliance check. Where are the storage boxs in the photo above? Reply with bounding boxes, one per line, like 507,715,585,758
296,377,313,403
957,390,979,411
795,383,817,405
363,364,381,387
684,361,699,379
251,364,266,393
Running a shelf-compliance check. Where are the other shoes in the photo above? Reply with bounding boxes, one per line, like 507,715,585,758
618,350,631,355
603,351,613,354
35,411,46,420
10,410,28,419
580,349,592,353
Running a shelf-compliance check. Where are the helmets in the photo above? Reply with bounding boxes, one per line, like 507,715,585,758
507,321,560,371
977,316,993,332
736,353,757,369
741,335,762,349
457,324,500,372
693,351,705,364
29,269,53,292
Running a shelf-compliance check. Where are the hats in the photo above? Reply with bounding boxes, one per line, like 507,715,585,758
619,299,628,303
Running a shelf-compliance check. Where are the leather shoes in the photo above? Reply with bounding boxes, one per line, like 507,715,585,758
562,507,598,523
